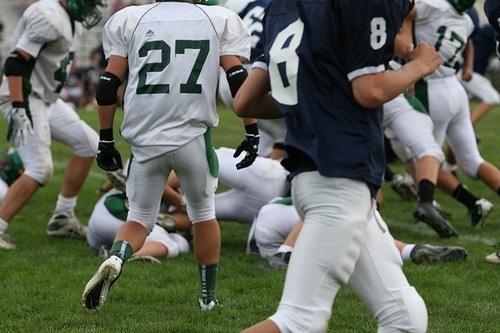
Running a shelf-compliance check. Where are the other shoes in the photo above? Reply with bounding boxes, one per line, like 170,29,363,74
98,244,110,260
126,256,161,265
485,252,500,263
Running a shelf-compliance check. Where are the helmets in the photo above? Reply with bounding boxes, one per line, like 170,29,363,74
67,0,106,28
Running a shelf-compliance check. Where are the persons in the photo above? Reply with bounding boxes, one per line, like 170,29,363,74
0,0,500,270
81,0,260,310
232,0,443,333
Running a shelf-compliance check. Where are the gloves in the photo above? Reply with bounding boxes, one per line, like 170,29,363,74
233,123,260,170
96,128,124,171
7,105,35,147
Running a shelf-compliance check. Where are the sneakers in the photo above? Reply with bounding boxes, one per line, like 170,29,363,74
124,199,178,231
409,243,468,264
469,198,493,229
198,297,219,315
106,170,127,192
440,147,457,177
411,203,460,239
81,256,124,314
46,207,89,239
272,249,291,266
0,232,18,250
389,174,416,202
